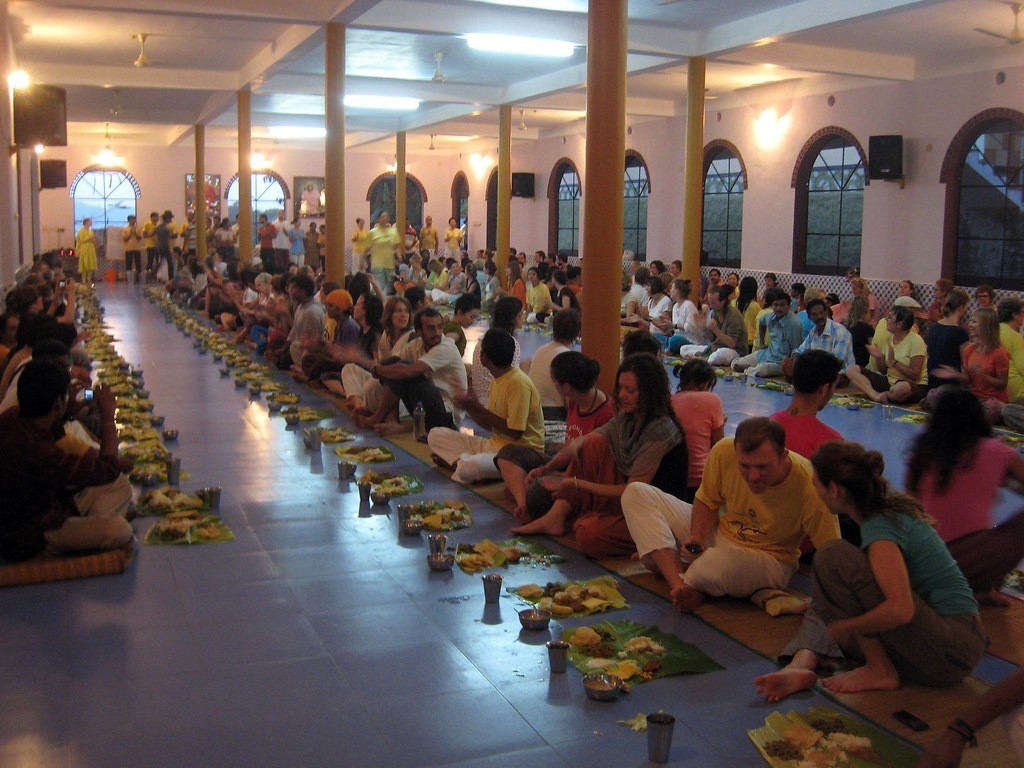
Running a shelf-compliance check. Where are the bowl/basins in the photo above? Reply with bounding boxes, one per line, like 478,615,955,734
348,463,357,476
581,673,624,701
402,518,424,535
371,492,391,505
519,609,552,630
426,553,455,571
196,486,210,503
64,279,325,485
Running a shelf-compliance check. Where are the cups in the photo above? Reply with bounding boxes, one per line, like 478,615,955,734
338,460,348,479
481,574,503,603
209,487,221,508
427,533,448,552
166,457,180,485
475,298,895,420
397,504,412,522
546,640,570,673
646,713,676,764
357,480,371,501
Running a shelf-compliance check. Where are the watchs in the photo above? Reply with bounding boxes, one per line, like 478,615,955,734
649,317,653,322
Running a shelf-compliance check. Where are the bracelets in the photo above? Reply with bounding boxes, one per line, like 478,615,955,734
373,366,379,376
574,475,578,494
890,361,898,367
675,324,677,329
947,717,978,749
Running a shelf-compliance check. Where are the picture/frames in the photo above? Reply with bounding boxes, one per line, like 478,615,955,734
292,175,326,218
184,174,222,219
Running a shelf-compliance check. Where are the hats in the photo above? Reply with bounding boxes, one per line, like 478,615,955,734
326,289,353,309
895,296,921,307
252,257,263,267
161,211,174,217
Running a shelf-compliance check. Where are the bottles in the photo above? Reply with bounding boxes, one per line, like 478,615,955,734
413,402,426,441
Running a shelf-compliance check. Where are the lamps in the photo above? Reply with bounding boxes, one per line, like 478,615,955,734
134,32,151,70
518,109,527,131
428,133,436,150
104,123,112,142
107,87,125,116
431,51,448,83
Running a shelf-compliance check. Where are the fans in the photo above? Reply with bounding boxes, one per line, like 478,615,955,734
971,2,1024,50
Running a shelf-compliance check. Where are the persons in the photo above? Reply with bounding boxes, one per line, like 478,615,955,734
898,279,917,298
894,296,923,334
302,182,322,214
931,306,1011,428
0,211,142,569
427,329,546,485
493,349,617,522
1003,390,1024,435
845,306,929,406
928,278,955,324
621,416,845,614
924,287,971,389
994,296,1024,401
767,348,861,566
508,352,689,558
141,208,881,434
668,357,728,503
900,384,1024,606
973,283,998,313
914,664,1024,768
753,441,991,703
